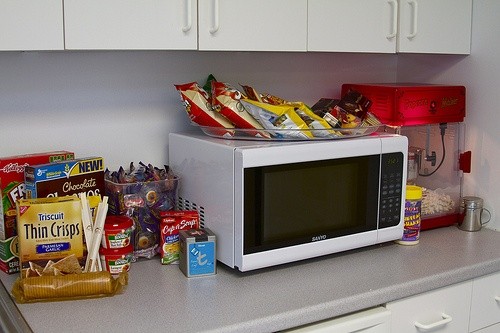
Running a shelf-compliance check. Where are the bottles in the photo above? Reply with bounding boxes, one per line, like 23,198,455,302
394,185,422,246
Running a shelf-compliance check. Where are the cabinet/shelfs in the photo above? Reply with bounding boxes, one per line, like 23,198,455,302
306,0,473,54
0,0,307,53
384,271,500,333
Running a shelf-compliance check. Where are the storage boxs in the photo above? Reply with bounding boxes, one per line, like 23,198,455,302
105,170,181,260
179,229,216,278
0,151,105,280
159,210,199,265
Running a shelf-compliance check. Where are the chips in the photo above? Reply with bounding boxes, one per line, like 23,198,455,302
26,254,81,277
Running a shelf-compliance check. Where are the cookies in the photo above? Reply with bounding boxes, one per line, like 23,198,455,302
22,271,112,300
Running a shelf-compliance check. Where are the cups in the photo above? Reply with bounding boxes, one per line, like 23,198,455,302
459,196,491,232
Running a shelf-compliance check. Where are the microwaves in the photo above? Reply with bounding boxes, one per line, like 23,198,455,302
167,131,409,274
